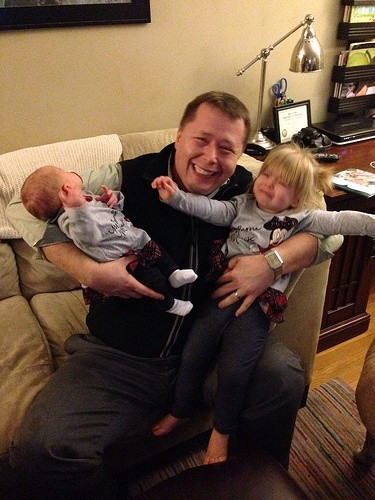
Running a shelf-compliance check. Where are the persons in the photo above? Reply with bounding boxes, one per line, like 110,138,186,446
152,141,375,466
6,91,344,500
20,165,198,316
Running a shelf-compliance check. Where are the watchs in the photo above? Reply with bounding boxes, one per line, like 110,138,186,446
264,249,284,281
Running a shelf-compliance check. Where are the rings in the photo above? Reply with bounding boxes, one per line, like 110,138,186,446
234,291,241,300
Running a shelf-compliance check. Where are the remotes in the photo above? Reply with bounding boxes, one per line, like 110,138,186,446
313,152,340,163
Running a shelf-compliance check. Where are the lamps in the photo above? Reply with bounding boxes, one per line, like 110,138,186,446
235,13,324,150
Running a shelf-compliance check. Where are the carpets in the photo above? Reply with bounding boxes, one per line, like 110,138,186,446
129,377,375,500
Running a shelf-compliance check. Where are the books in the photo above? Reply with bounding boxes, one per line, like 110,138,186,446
342,4,375,23
334,41,375,99
330,167,375,198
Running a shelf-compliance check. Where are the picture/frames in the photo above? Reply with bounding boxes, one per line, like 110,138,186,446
272,100,311,143
0,0,151,31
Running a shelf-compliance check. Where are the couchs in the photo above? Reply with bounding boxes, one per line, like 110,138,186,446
0,127,331,500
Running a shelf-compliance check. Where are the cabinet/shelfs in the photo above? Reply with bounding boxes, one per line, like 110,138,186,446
254,139,375,352
322,0,375,135
